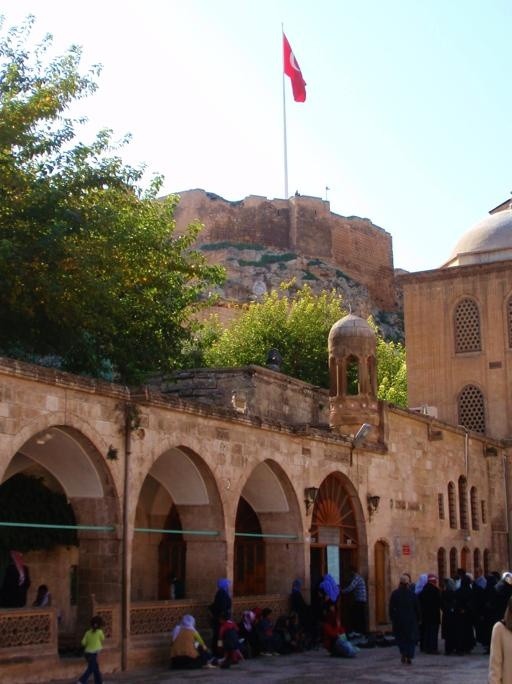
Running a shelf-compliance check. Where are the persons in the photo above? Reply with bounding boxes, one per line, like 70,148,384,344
170,609,315,669
4,548,32,607
289,567,368,657
208,577,232,644
488,600,511,684
79,611,107,680
33,582,63,627
388,566,512,663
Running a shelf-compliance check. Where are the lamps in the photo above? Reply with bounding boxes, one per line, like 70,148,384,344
304,485,319,516
367,495,380,523
428,425,444,440
484,441,498,456
354,423,372,441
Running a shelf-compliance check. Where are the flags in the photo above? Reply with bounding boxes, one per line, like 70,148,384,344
282,33,306,101
